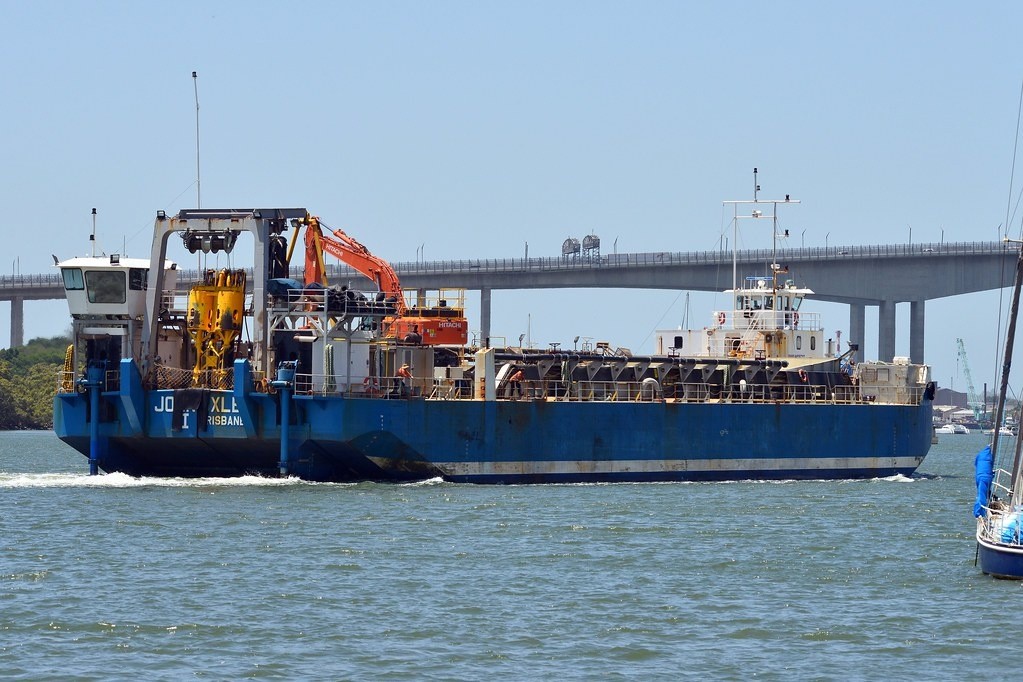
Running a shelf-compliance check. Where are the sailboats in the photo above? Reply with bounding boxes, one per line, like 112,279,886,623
943,377,971,435
971,227,1022,583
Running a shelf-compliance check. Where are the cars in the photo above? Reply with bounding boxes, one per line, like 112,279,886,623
923,248,934,252
839,251,849,255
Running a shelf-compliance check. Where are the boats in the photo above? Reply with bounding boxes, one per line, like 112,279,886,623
53,68,942,486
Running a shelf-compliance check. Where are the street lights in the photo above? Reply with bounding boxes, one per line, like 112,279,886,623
825,232,830,258
907,224,912,253
801,229,807,256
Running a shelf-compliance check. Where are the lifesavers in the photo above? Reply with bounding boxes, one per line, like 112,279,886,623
793,312,799,326
799,369,808,383
720,312,726,325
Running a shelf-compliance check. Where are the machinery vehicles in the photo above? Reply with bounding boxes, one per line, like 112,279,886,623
281,215,470,349
